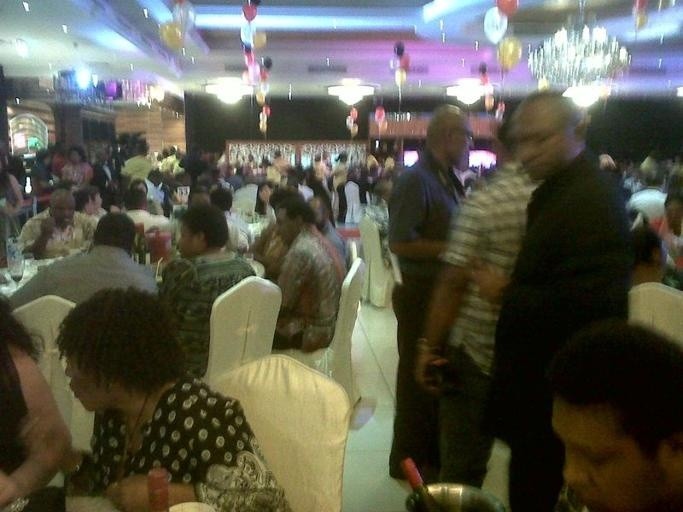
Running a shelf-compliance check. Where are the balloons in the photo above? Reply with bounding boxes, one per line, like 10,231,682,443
631,0,678,33
482,6,508,44
239,2,274,136
496,34,522,71
494,0,520,18
158,0,196,55
478,60,506,121
345,104,386,139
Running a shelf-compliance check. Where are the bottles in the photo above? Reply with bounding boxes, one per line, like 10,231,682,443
137,223,147,265
400,456,445,512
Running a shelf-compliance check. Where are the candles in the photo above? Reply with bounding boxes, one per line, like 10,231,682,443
593,28,600,41
560,29,567,41
620,49,626,61
554,33,560,45
582,28,589,41
600,28,607,40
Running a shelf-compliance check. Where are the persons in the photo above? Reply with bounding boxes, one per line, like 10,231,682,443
0,288,73,512
627,154,668,225
473,90,632,512
0,135,393,219
7,211,158,311
415,117,544,490
650,176,683,290
20,286,292,511
158,203,256,378
273,199,347,351
389,41,410,89
388,103,474,474
18,187,98,261
546,320,683,512
628,226,683,348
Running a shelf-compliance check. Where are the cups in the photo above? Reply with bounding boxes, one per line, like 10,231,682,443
7,238,25,290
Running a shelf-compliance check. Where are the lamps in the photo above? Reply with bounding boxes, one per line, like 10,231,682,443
327,77,379,106
442,79,496,107
200,77,258,104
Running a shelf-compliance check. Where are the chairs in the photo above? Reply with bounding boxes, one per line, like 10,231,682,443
204,356,351,507
628,282,683,345
204,276,283,376
358,214,394,308
285,257,366,408
344,181,362,223
9,293,77,460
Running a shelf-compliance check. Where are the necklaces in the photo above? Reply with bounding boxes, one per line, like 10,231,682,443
123,394,150,457
